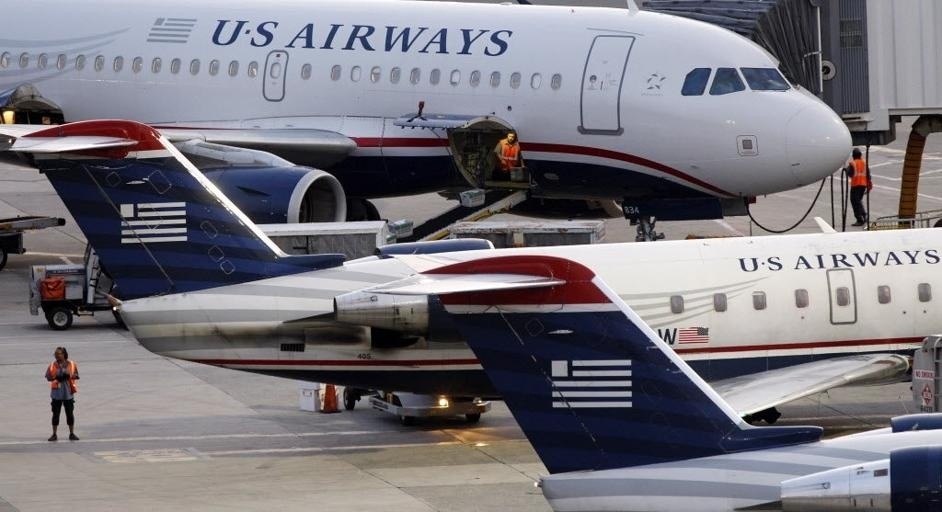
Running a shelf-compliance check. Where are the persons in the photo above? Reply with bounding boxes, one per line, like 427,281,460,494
845,148,872,226
45,346,80,442
492,131,522,181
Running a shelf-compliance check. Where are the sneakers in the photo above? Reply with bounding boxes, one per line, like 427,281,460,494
854,215,867,226
70,434,79,440
48,434,57,441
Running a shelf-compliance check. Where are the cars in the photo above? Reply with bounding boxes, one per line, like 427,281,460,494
342,387,493,427
29,240,127,331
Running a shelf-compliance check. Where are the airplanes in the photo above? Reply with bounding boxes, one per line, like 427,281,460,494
334,254,942,511
1,1,853,241
0,117,941,420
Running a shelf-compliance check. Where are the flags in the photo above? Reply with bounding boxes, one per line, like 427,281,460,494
678,327,709,344
